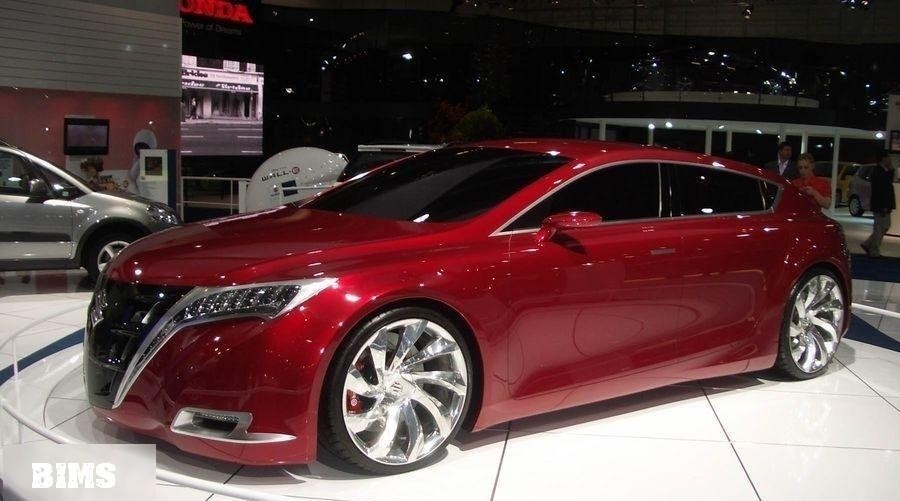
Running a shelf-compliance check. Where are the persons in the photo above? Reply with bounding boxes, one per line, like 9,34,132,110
792,154,833,212
764,141,796,181
860,151,897,257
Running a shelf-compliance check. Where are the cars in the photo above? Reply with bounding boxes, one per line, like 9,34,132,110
1,145,183,285
87,138,853,475
848,162,878,216
814,160,862,207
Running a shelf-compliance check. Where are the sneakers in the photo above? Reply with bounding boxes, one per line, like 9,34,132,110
860,243,882,258
